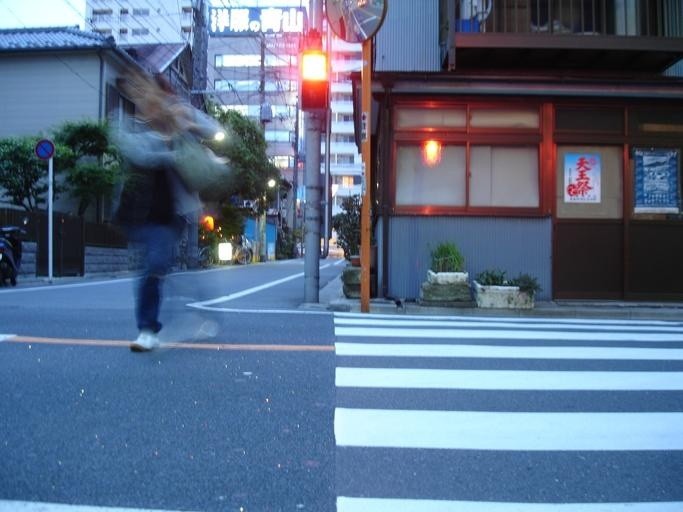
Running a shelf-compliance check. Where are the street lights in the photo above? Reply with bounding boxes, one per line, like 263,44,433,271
248,21,266,259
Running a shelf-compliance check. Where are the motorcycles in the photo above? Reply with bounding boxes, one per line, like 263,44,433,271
0,216,29,286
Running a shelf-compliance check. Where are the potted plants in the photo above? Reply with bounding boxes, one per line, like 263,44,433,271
346,243,362,267
472,270,545,309
358,222,377,273
425,241,469,286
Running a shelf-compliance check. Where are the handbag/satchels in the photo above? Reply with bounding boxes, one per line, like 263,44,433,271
118,172,172,224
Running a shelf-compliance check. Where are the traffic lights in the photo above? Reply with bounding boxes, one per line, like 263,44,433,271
298,52,329,109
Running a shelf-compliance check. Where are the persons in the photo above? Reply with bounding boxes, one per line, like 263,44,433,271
106,63,220,352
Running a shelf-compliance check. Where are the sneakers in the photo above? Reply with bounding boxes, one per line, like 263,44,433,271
132,331,160,350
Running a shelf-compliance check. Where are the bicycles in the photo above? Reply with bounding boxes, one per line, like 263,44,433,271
177,241,253,269
